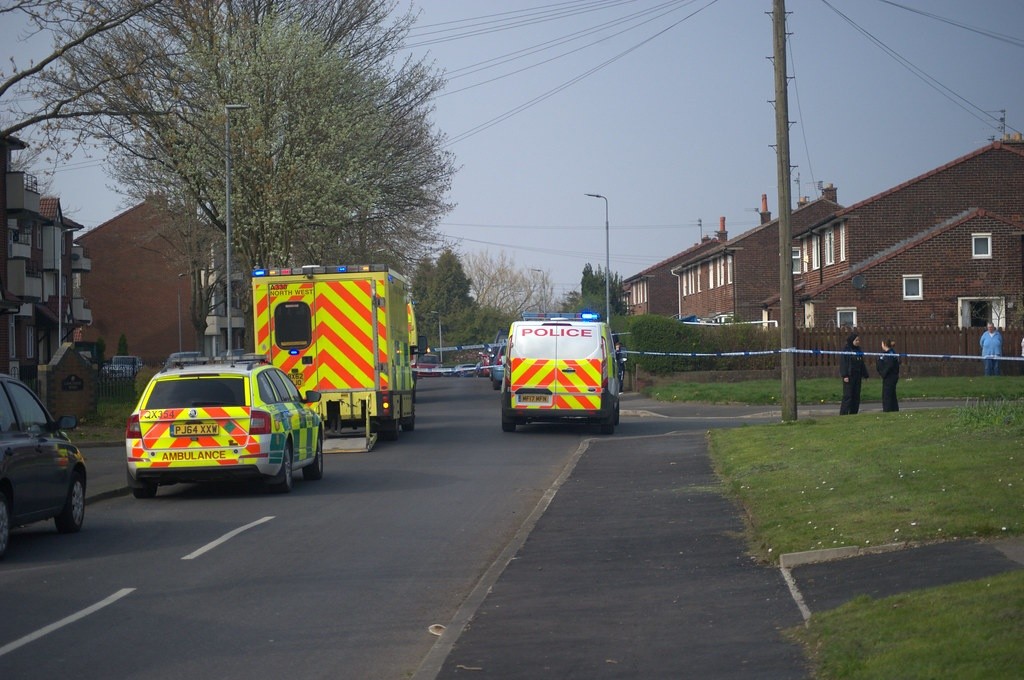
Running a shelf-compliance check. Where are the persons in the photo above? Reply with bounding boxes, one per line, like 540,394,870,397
980,322,1003,377
615,342,624,396
839,332,869,415
876,336,899,412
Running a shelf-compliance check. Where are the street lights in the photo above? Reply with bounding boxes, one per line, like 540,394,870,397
225,101,248,359
584,193,611,325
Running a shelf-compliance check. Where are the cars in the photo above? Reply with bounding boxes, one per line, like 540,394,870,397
100,350,249,377
417,330,511,390
0,373,88,555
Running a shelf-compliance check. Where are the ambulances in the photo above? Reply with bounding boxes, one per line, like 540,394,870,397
252,266,420,442
501,312,620,434
126,349,324,498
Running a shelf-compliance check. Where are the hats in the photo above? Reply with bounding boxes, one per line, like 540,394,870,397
847,333,860,342
